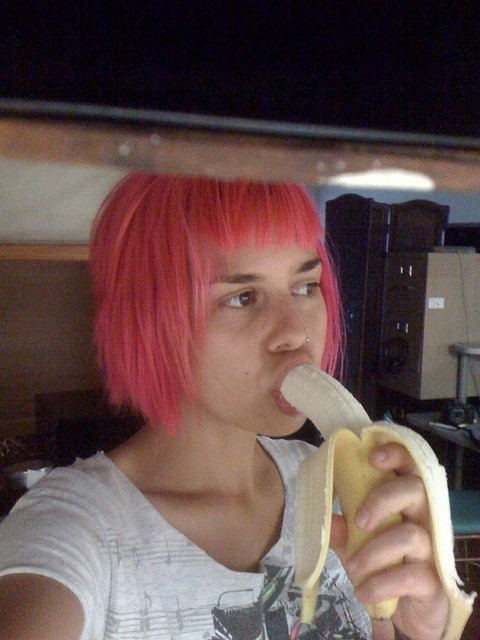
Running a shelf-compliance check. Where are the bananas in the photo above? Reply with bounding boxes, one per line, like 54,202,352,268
280,361,477,640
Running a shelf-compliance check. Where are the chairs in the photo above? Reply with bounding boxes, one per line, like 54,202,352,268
383,411,480,562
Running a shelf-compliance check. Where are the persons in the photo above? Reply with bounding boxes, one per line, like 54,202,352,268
0,169,448,640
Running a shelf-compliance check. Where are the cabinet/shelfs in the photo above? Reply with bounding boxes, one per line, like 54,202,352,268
405,344,480,491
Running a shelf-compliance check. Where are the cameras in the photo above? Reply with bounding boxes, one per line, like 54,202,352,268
443,399,475,427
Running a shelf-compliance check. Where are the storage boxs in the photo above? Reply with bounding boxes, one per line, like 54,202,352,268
377,252,480,401
